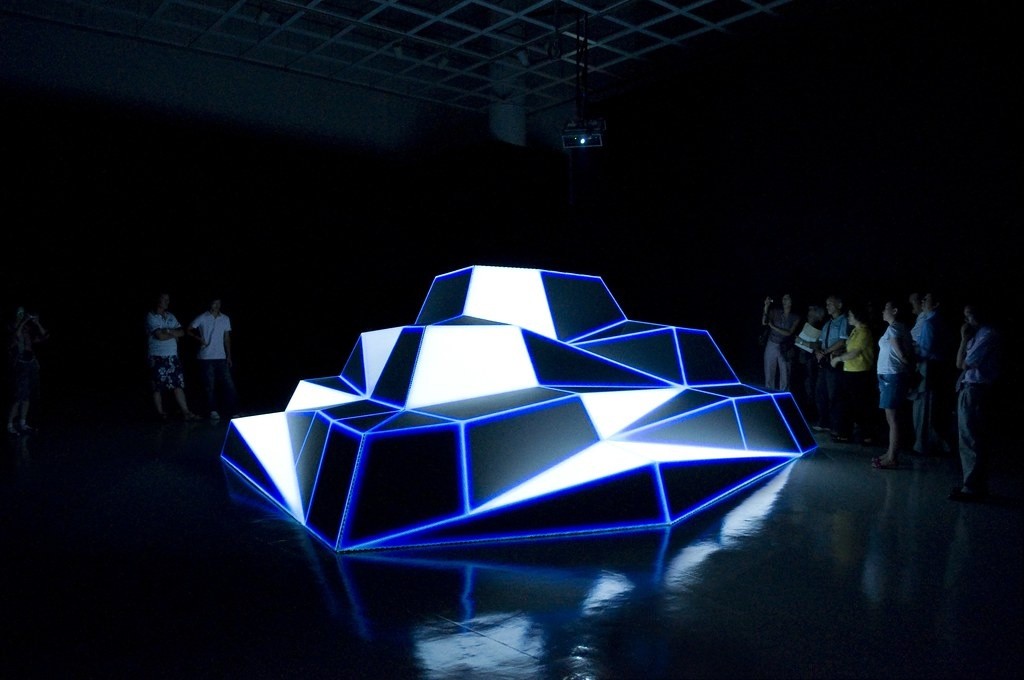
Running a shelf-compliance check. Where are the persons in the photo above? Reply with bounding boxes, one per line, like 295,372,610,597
761,293,799,391
948,300,999,502
6,305,49,437
187,296,242,422
798,303,828,413
907,291,925,324
146,287,202,421
871,298,909,467
810,294,849,436
909,291,942,465
831,306,874,445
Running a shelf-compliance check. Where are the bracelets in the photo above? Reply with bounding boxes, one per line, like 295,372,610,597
166,328,170,334
799,340,803,345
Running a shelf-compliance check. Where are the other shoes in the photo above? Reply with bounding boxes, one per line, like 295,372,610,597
950,487,963,492
209,411,220,419
185,413,203,421
19,423,38,432
813,426,830,430
832,437,854,442
948,493,967,501
5,423,20,436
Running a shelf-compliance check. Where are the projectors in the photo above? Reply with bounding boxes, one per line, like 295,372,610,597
561,117,606,148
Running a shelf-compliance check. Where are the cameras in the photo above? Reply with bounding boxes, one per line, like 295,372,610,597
769,300,773,303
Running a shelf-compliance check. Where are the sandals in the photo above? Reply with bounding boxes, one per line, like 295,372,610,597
872,459,897,469
871,457,880,461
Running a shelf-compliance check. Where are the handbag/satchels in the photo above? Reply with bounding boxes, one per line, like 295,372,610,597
759,324,769,352
821,354,830,368
18,353,41,372
828,359,843,372
